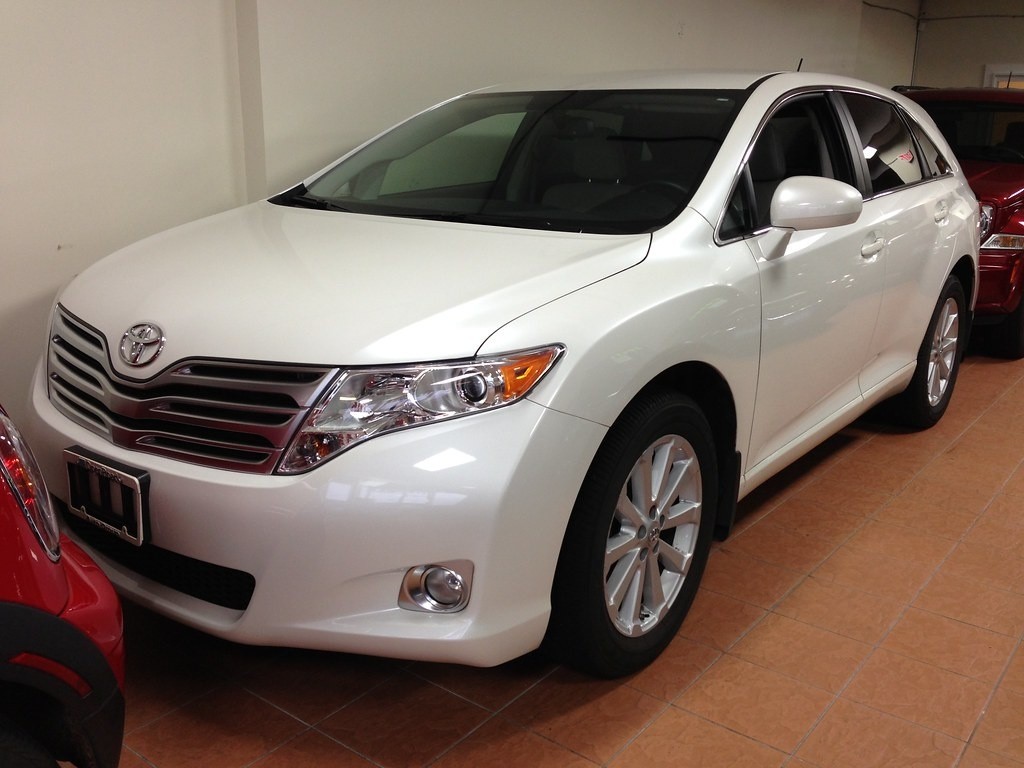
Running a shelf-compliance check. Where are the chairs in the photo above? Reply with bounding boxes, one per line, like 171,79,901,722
1005,122,1024,156
732,122,785,228
543,125,626,212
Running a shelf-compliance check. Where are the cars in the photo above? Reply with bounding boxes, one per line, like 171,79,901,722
24,67,983,675
0,401,126,768
882,81,1024,331
